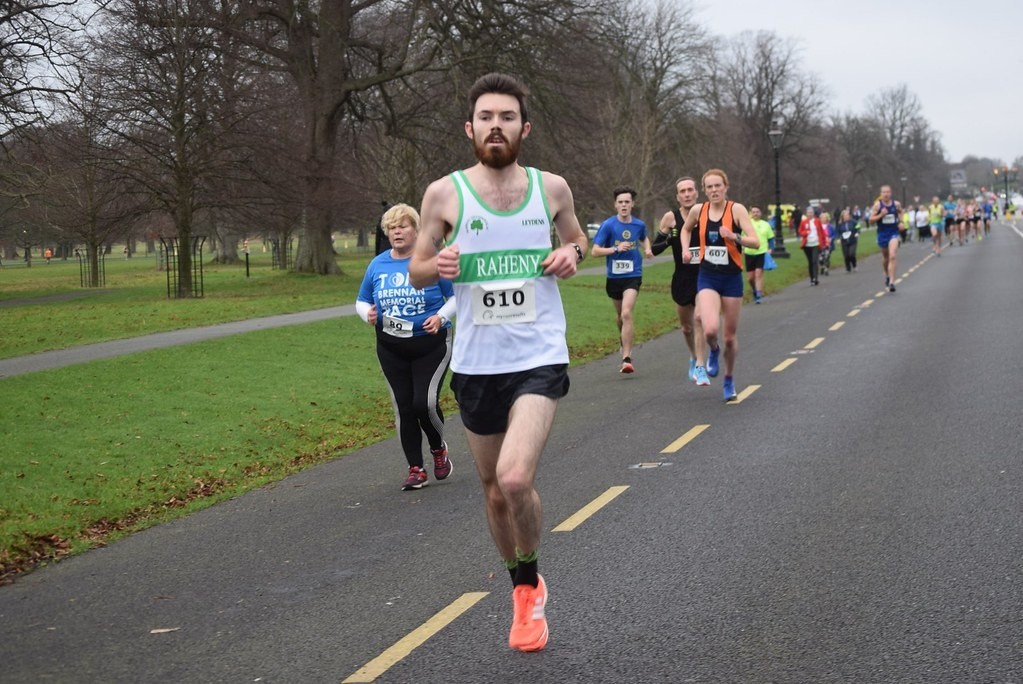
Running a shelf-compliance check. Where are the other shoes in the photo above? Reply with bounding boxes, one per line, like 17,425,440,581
756,291,762,304
752,290,757,301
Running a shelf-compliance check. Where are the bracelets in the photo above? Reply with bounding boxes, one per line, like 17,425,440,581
613,244,619,253
568,243,584,262
735,234,740,243
436,313,446,328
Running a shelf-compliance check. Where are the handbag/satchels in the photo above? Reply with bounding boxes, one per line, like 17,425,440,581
763,252,776,271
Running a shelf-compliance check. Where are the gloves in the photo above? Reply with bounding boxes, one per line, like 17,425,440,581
664,228,679,244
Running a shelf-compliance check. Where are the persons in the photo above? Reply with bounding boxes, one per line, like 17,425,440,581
992,199,999,221
409,73,588,652
680,168,759,400
742,205,777,301
798,206,836,287
902,195,945,257
943,194,993,248
651,176,699,381
788,203,803,241
869,185,904,291
355,203,456,491
1004,200,1016,225
837,205,870,274
591,185,654,374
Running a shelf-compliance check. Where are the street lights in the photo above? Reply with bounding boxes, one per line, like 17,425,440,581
841,183,850,207
993,165,1017,214
901,172,908,207
766,118,790,259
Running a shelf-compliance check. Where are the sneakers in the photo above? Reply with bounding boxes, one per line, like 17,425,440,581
722,376,737,400
401,466,429,491
695,366,711,386
619,362,634,374
688,357,697,381
509,571,548,649
620,345,632,358
429,440,453,481
518,628,549,652
706,344,720,377
